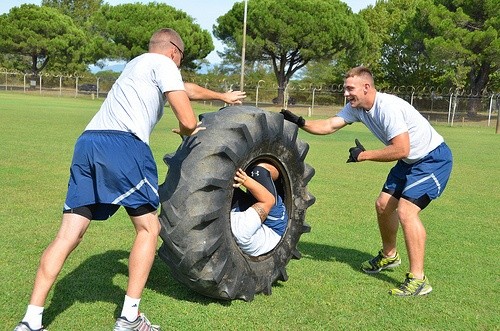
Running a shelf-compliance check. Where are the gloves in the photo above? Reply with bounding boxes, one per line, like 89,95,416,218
280,109,305,127
346,138,366,163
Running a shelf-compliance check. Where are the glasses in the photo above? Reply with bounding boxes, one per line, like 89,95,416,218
170,41,185,65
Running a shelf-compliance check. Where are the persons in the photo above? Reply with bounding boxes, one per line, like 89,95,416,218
229,163,288,259
11,27,246,331
279,67,452,297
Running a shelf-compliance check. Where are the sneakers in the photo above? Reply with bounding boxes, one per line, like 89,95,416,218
360,249,401,274
112,311,160,331
13,321,48,331
387,273,432,297
